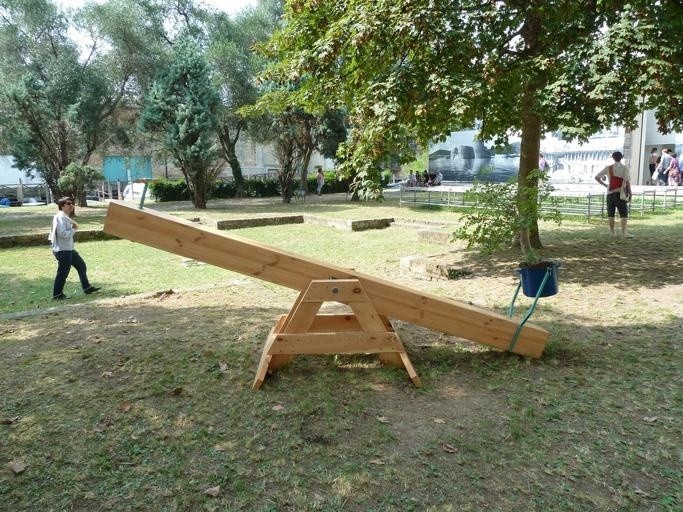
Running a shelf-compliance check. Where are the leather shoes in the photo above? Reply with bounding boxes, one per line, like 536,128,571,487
85,286,101,295
50,293,71,300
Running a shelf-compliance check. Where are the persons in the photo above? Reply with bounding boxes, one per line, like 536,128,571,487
403,168,443,188
315,168,325,197
45,195,103,300
538,153,546,185
645,147,683,188
594,151,635,240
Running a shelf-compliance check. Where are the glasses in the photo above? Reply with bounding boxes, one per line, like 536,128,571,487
62,201,75,205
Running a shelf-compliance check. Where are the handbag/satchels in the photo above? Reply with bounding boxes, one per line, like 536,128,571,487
620,166,631,202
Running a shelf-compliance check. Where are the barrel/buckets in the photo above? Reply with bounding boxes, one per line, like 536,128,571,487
516,259,558,298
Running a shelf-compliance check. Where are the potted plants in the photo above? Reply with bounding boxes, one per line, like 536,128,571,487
445,169,562,298
